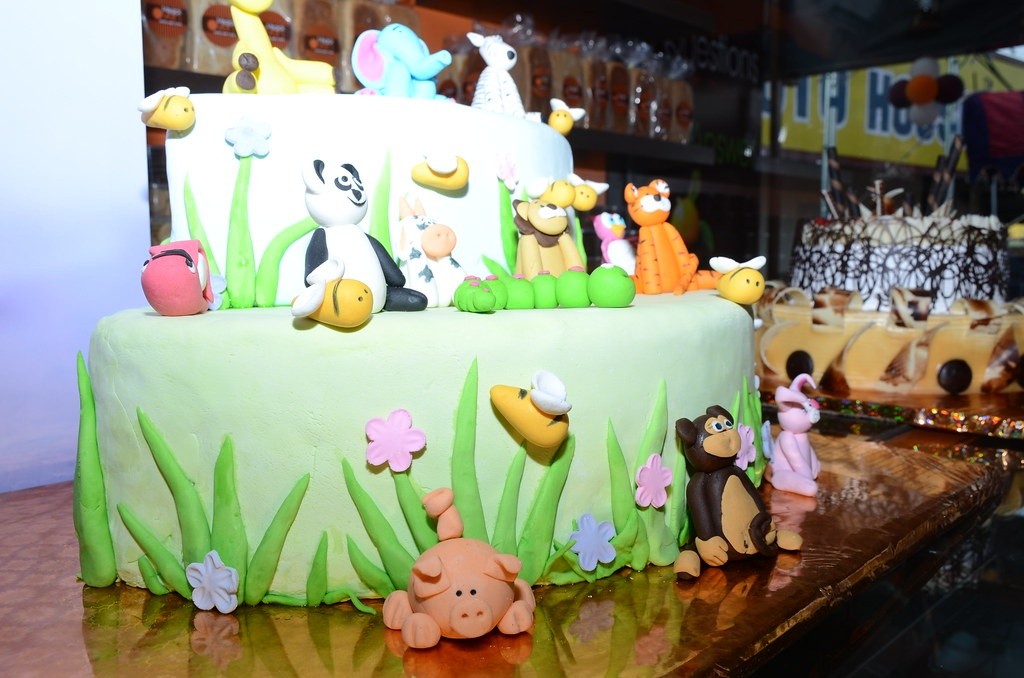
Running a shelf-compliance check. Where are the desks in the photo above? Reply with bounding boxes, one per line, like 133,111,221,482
0,427,1010,677
759,379,1024,522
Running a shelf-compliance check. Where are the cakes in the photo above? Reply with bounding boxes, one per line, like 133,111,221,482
72,0,822,650
753,131,1024,395
